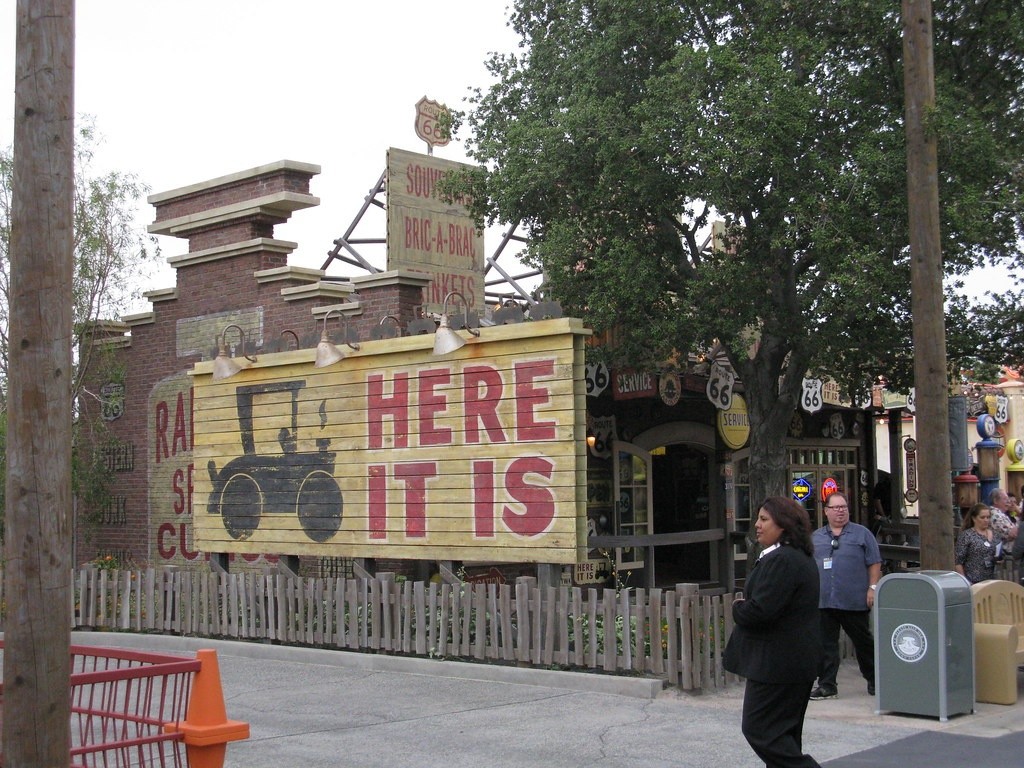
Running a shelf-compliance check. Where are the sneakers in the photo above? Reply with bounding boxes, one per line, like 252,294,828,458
865,678,876,695
810,685,839,699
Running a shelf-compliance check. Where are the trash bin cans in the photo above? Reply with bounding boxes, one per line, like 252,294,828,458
872,567,978,721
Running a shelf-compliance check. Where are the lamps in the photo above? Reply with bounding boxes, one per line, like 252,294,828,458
429,291,481,356
314,309,360,369
211,324,256,378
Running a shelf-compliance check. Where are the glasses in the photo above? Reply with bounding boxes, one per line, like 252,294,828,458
825,502,849,511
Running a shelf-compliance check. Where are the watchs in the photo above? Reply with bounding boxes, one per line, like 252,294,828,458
868,585,876,590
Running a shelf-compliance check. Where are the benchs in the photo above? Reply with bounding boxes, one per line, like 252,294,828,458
972,579,1024,705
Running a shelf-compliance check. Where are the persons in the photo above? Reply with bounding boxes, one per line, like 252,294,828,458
722,497,825,768
809,491,884,701
988,487,1018,556
1018,485,1024,513
1006,492,1019,524
955,503,1001,585
